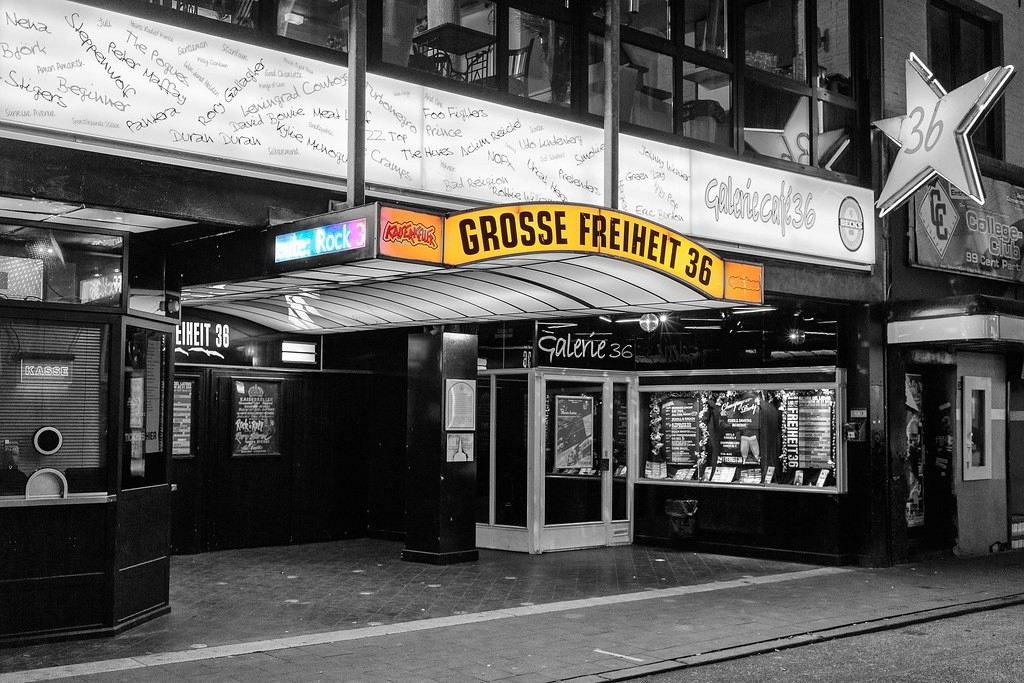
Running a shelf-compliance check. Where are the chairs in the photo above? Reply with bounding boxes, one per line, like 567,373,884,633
469,37,535,98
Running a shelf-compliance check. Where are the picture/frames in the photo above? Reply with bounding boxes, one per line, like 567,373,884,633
230,375,284,458
554,395,594,469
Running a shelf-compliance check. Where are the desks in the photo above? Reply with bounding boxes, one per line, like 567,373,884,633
413,21,496,87
